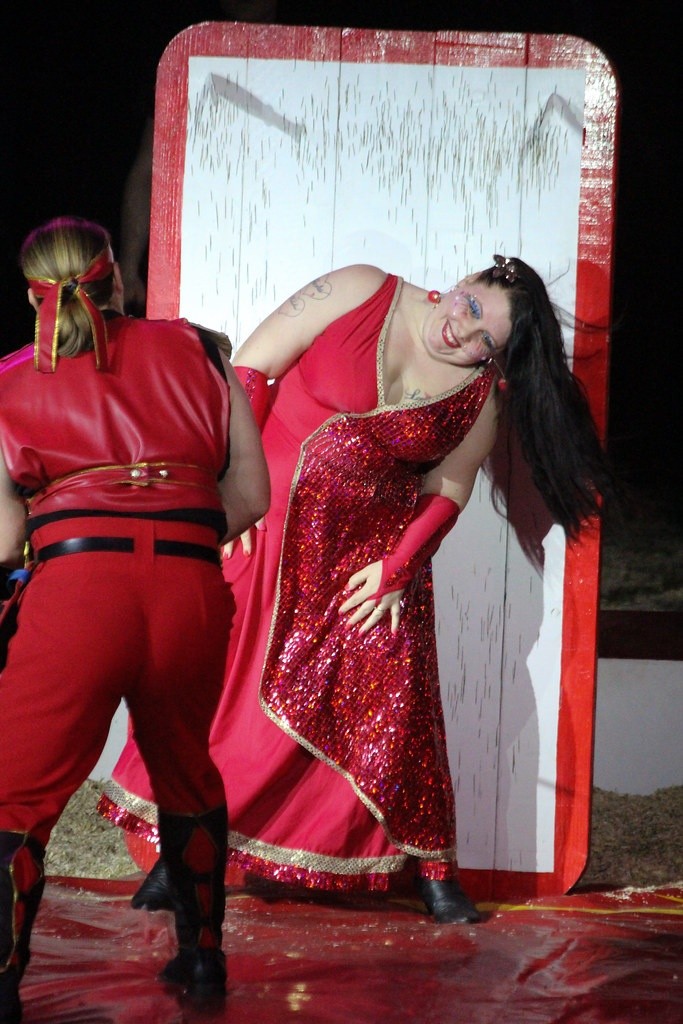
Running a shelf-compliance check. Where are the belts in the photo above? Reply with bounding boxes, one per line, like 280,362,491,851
36,537,223,567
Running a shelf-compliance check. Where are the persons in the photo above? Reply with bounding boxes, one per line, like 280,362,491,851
98,253,614,924
0,216,273,1024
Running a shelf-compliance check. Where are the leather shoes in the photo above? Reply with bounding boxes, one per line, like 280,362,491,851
416,875,482,925
131,855,173,912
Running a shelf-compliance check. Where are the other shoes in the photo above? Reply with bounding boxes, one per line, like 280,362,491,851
157,947,229,1013
0,971,25,1024
